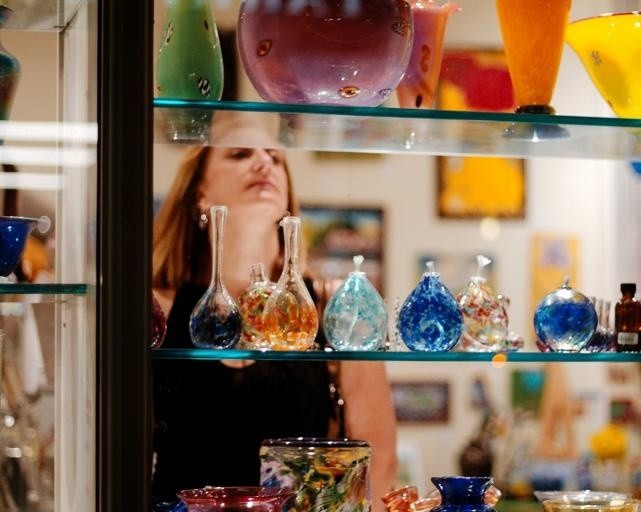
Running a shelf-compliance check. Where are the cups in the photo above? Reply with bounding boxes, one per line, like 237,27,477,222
384,0,462,148
495,0,574,140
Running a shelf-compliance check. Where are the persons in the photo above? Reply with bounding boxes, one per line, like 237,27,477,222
147,116,402,512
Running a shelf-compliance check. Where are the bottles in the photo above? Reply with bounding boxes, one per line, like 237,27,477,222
389,293,525,354
533,277,606,353
322,255,389,353
192,206,239,352
263,215,318,352
397,261,465,350
457,255,508,353
237,264,275,353
614,281,641,352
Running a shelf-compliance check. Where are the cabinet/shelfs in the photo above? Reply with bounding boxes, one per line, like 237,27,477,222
127,1,641,511
0,0,127,510
